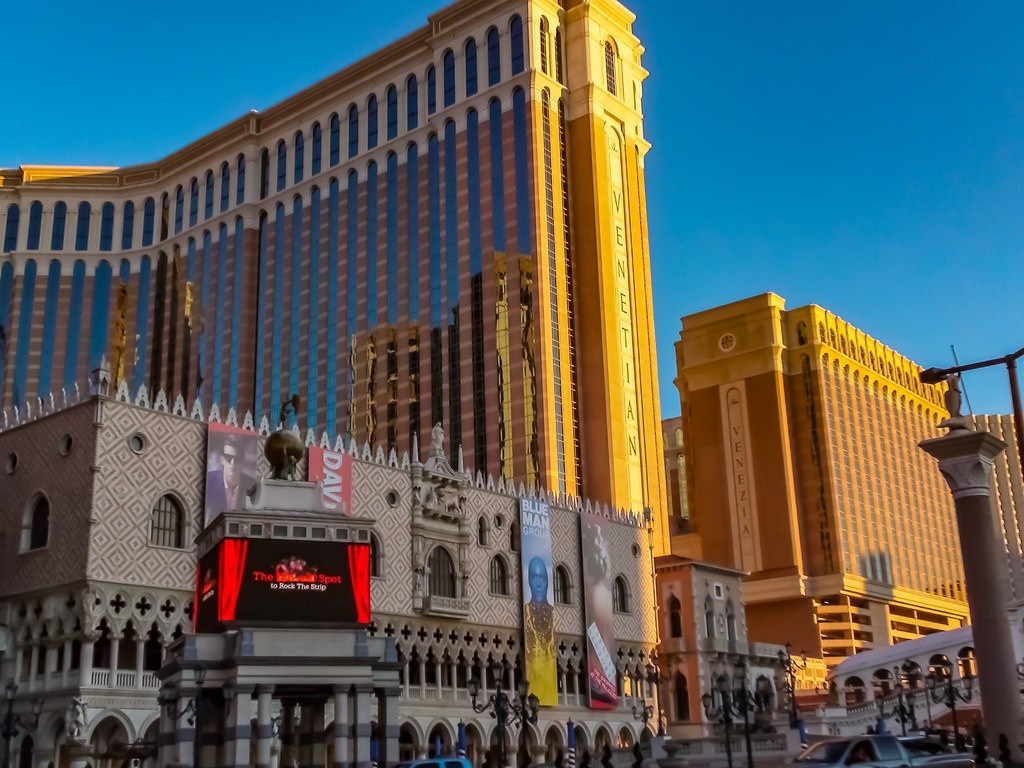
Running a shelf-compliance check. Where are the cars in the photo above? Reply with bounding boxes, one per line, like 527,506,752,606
897,735,958,757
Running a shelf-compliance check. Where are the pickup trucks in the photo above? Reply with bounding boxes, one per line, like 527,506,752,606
784,734,977,768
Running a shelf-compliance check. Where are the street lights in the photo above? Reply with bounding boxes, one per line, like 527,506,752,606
776,641,808,713
917,347,1024,486
270,707,302,768
875,681,918,736
924,657,975,735
465,660,540,767
631,699,655,728
156,661,237,768
642,655,673,736
0,680,45,768
702,670,760,767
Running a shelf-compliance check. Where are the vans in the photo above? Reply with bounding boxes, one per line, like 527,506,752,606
390,755,474,768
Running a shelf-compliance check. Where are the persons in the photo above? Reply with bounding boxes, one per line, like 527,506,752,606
282,448,296,481
278,398,294,430
585,525,613,694
206,439,255,521
525,557,556,682
431,422,444,457
